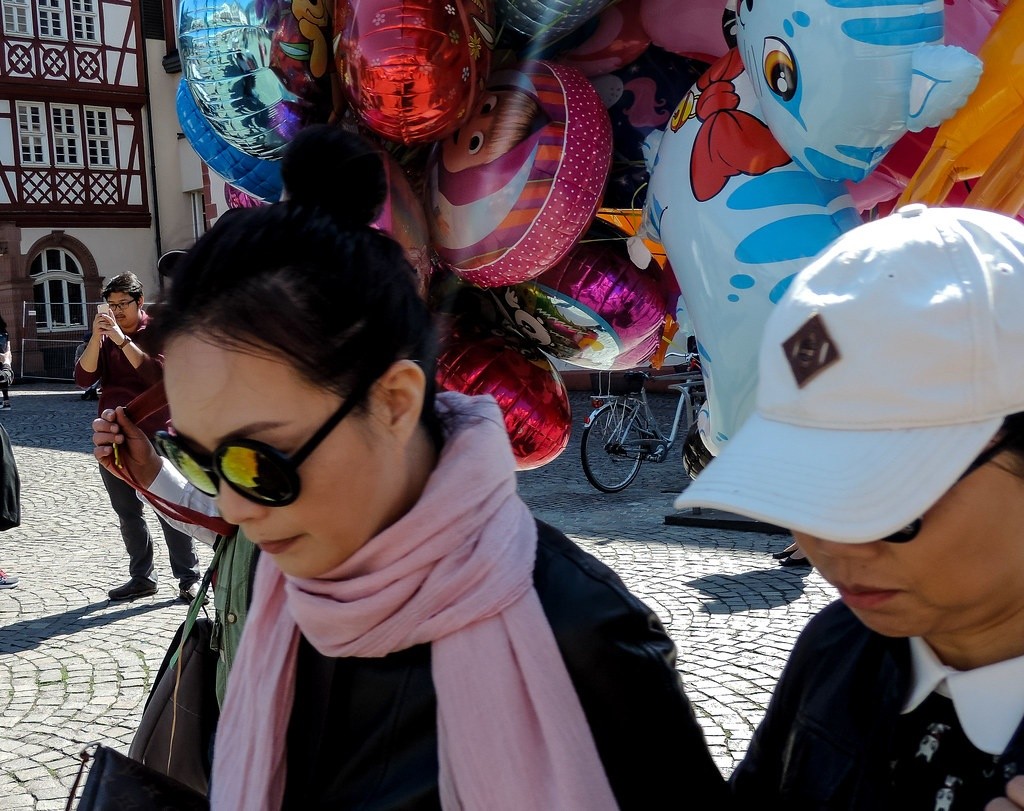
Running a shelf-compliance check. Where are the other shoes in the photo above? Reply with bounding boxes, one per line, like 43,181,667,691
772,548,797,559
779,555,812,566
80,389,99,402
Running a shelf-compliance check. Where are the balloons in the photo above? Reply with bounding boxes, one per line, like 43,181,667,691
173,0,1024,468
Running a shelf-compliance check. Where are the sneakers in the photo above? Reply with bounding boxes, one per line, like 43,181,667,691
0,404,11,410
0,569,18,588
180,578,210,606
108,578,159,599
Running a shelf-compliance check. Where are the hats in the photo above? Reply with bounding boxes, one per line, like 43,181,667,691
83,333,92,341
674,203,1023,542
157,250,193,280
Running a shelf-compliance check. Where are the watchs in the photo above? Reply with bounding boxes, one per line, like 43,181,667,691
117,335,132,349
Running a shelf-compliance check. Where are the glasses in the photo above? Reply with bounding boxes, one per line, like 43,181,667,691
154,359,423,508
880,425,1016,542
109,298,137,311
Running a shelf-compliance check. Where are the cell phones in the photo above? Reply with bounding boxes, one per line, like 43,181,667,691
96,304,109,315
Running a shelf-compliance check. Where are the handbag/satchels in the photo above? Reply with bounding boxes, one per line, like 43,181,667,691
63,741,211,811
127,534,222,799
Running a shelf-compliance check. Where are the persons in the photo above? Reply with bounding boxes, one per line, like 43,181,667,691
72,269,211,606
0,315,12,412
0,342,19,589
773,538,813,568
141,203,726,810
676,203,1024,811
92,407,227,546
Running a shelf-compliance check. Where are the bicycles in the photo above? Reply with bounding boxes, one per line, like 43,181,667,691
581,353,707,493
